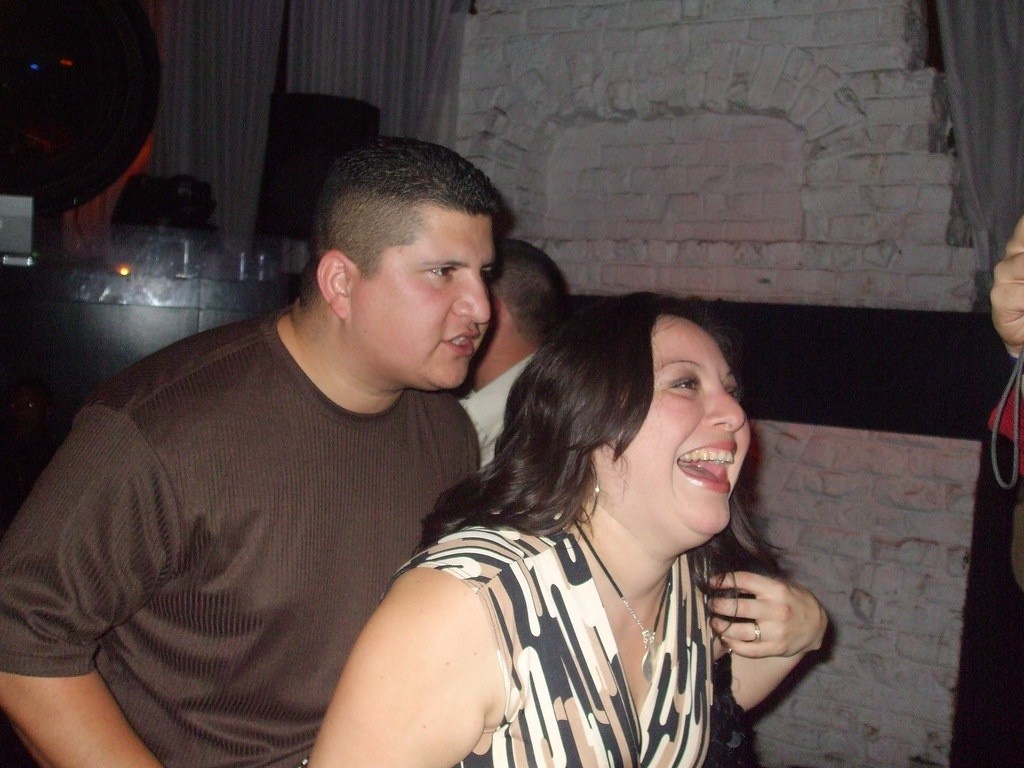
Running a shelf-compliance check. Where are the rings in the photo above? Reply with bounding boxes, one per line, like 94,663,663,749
753,620,761,644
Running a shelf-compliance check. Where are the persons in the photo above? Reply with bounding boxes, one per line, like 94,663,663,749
950,212,1023,768
0,139,830,768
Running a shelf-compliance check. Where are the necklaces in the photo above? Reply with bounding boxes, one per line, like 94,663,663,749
575,515,670,684
